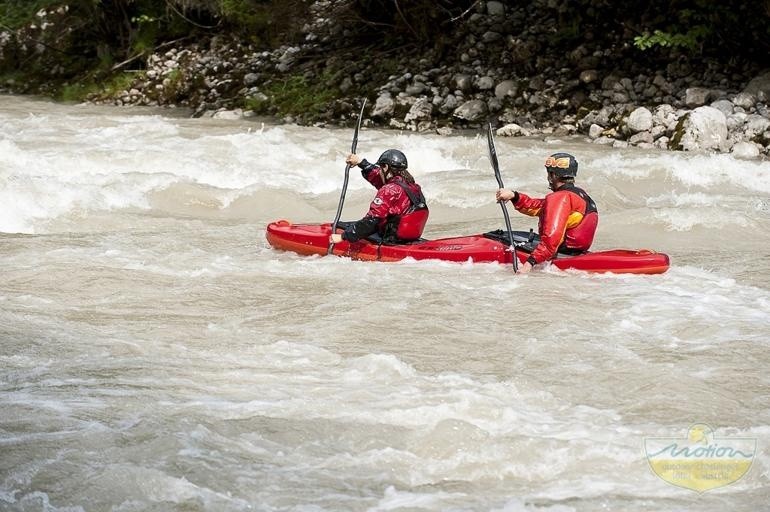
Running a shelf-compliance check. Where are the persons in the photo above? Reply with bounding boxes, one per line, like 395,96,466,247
329,149,429,246
496,153,598,273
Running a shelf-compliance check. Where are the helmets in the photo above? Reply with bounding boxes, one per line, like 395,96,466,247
376,149,407,169
545,153,578,179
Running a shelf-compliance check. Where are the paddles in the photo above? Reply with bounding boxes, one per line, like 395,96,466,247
328,97,367,254
486,122,519,272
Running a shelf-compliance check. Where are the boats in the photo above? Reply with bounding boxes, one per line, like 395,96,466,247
265,219,672,273
